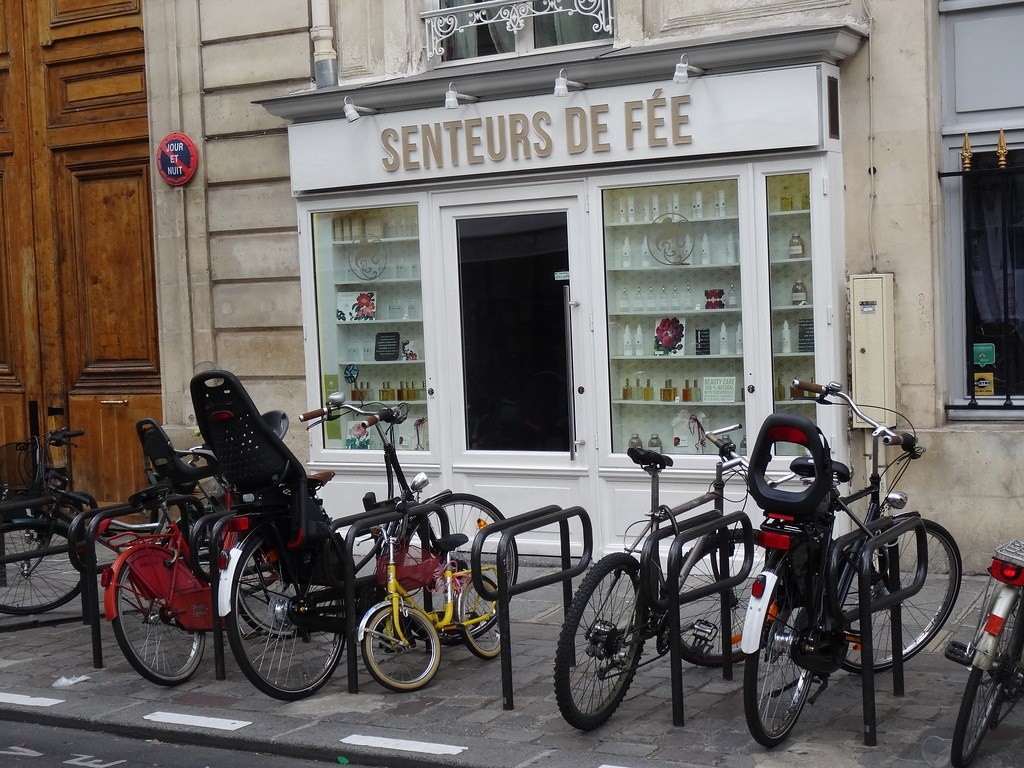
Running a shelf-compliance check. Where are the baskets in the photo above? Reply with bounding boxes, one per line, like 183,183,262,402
0,442,44,488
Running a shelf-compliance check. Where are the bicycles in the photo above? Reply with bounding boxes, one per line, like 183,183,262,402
945,540,1024,768
189,363,518,700
357,499,500,693
553,423,770,731
1,419,338,687
741,375,963,748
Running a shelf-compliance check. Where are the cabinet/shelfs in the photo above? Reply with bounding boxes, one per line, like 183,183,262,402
292,183,442,464
586,150,847,482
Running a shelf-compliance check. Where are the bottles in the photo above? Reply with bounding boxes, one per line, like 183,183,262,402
792,279,807,305
780,186,792,212
801,186,809,210
647,433,661,454
788,232,806,259
721,434,732,444
740,436,747,456
628,434,642,449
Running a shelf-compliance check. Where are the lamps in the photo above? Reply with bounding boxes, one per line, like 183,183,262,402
673,54,705,83
343,96,384,123
554,68,586,97
444,81,480,110
